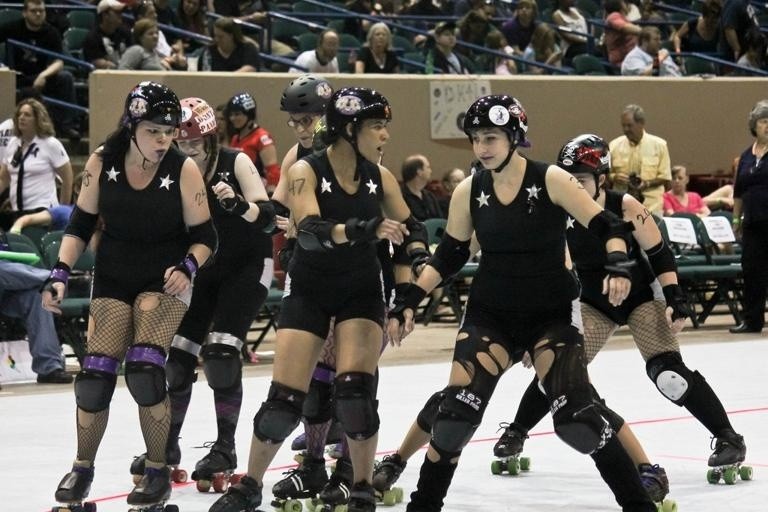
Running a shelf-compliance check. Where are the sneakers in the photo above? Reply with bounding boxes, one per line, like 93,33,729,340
37,370,73,383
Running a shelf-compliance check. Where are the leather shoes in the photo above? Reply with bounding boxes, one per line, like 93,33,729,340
730,322,762,333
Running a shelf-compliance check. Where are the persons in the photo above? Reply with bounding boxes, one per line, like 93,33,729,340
40,77,753,511
0,1,768,384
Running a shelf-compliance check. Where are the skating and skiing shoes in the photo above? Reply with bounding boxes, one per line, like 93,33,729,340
639,463,676,512
492,423,529,475
128,438,263,512
707,430,752,484
271,420,406,512
52,459,96,512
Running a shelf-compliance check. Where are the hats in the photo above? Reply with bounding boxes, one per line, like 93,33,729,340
435,22,456,32
97,0,126,14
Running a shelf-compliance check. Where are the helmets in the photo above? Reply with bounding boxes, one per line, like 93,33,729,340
228,94,256,120
280,76,391,137
464,95,528,146
123,81,216,141
557,134,611,174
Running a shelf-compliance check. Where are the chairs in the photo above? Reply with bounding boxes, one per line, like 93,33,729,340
0,1,767,375
